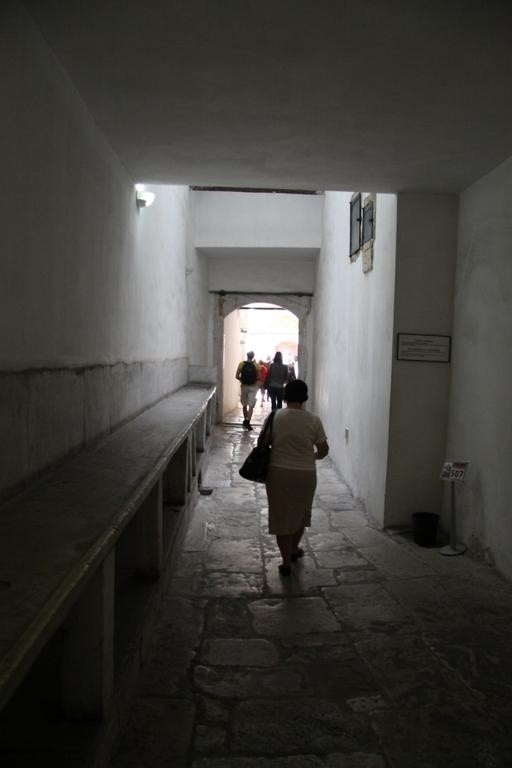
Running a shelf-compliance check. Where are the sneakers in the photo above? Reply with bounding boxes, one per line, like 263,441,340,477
243,420,253,430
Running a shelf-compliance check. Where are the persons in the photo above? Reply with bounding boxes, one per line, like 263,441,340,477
234,351,295,430
258,378,329,577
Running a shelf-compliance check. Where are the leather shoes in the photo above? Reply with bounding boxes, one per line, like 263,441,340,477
279,549,303,576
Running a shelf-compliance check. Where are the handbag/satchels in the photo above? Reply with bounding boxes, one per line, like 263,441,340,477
239,447,272,483
265,376,271,384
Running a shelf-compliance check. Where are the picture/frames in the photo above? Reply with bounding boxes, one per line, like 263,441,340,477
397,331,452,364
348,192,375,275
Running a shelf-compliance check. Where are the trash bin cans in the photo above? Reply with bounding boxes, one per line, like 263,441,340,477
412,512,440,548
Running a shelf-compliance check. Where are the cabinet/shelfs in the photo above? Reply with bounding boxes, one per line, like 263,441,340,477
1,381,221,719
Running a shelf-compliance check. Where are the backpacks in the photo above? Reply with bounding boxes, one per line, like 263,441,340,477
241,361,256,385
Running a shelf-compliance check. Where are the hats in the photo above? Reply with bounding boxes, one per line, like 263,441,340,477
284,380,308,402
248,351,254,358
